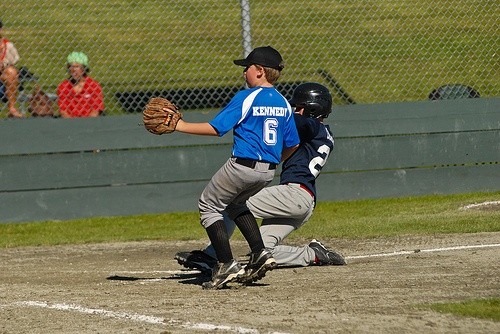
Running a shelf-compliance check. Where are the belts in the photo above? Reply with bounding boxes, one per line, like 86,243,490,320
285,182,314,198
233,158,277,171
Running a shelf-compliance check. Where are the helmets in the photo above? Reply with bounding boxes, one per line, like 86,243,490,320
287,82,334,121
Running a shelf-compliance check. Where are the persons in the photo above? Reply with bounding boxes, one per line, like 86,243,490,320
143,46,300,291
56,52,104,117
0,22,26,119
173,83,348,274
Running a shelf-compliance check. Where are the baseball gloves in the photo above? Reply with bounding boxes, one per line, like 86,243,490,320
143,98,181,135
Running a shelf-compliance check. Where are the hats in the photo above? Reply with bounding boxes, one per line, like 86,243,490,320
232,46,285,71
66,51,87,67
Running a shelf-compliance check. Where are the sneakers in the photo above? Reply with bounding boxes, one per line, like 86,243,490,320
237,248,278,286
202,258,246,290
309,238,345,266
174,249,220,279
